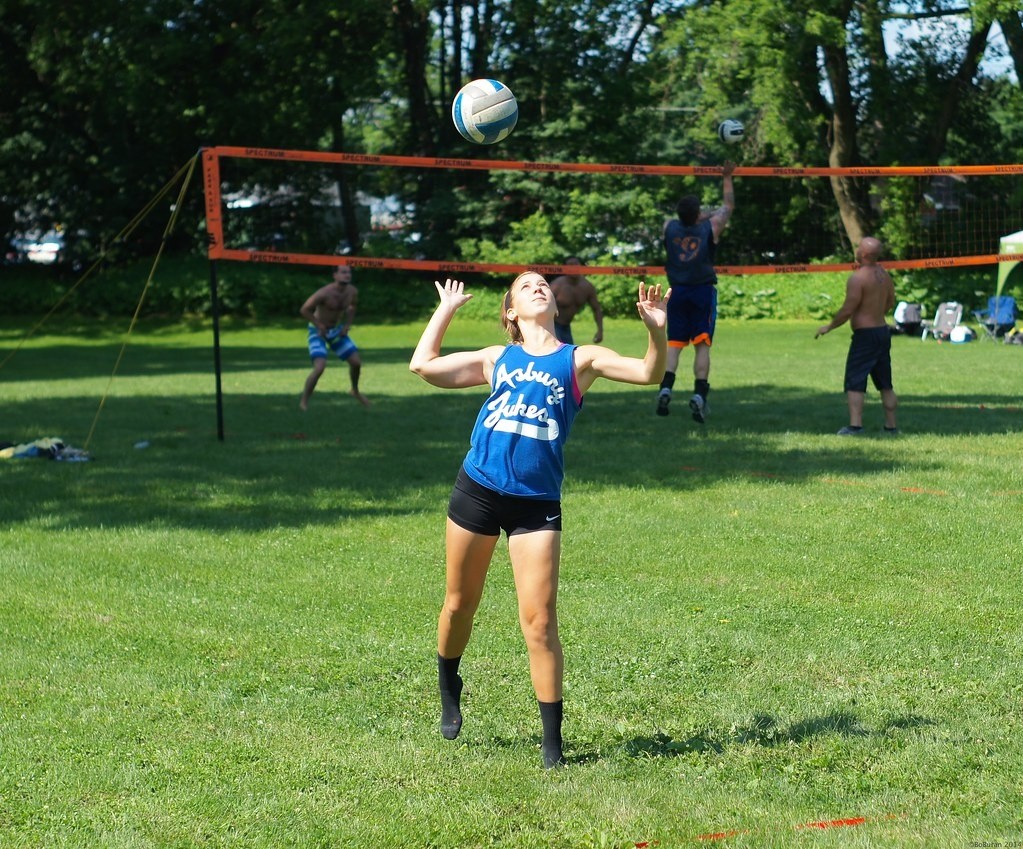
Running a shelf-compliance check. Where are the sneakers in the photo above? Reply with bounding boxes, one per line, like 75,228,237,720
656,388,671,416
689,394,705,424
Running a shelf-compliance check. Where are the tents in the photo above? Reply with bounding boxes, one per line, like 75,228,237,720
995,231,1023,343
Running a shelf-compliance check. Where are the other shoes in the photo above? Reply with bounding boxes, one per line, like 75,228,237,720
879,427,899,437
837,426,863,435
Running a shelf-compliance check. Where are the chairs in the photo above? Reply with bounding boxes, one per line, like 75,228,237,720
975,293,1015,346
922,302,963,346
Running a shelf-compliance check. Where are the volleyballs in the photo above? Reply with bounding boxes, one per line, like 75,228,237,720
717,119,746,146
450,78,519,145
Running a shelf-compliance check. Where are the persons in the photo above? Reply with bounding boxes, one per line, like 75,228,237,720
301,266,371,412
550,257,603,344
815,237,897,435
409,270,672,773
657,161,737,424
